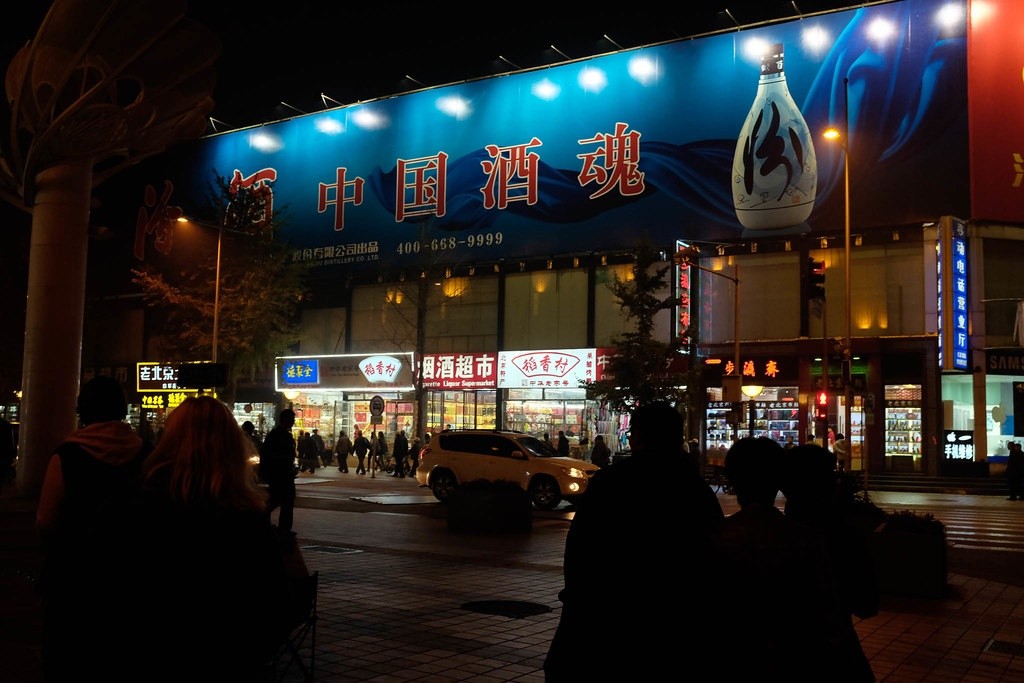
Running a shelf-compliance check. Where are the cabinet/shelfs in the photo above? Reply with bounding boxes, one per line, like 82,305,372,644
850,410,866,436
886,418,922,455
706,416,798,445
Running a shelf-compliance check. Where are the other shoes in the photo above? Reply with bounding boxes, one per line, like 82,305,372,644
343,470,348,473
366,470,370,472
356,470,358,474
1006,495,1017,500
1017,495,1024,500
360,472,365,474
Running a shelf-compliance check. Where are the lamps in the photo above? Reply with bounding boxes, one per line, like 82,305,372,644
377,229,903,282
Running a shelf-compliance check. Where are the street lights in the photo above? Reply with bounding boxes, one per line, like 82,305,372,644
741,379,765,438
282,390,301,433
822,128,852,501
178,215,255,400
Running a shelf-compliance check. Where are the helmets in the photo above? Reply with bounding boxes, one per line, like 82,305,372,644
413,436,421,442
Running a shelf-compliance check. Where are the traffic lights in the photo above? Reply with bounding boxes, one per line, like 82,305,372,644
804,255,826,300
815,391,829,421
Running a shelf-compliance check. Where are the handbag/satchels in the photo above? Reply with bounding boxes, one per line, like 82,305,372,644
376,443,381,452
362,439,369,447
390,457,397,464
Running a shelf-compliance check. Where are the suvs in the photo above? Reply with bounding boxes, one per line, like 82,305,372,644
416,428,601,512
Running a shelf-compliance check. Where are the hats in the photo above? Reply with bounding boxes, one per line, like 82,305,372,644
689,438,698,443
78,376,128,419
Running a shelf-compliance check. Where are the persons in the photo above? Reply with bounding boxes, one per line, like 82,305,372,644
139,420,163,448
242,408,297,536
445,424,451,432
87,397,309,683
0,417,14,492
392,428,436,479
682,436,700,464
298,429,335,474
335,431,388,475
543,400,724,683
542,433,554,448
680,438,825,683
778,445,880,683
591,435,611,469
557,431,570,457
1006,442,1024,501
784,434,846,472
35,376,147,683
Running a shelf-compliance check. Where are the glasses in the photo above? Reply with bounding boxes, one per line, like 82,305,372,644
625,430,632,438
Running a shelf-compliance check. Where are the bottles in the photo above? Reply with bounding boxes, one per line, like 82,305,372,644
731,42,817,230
